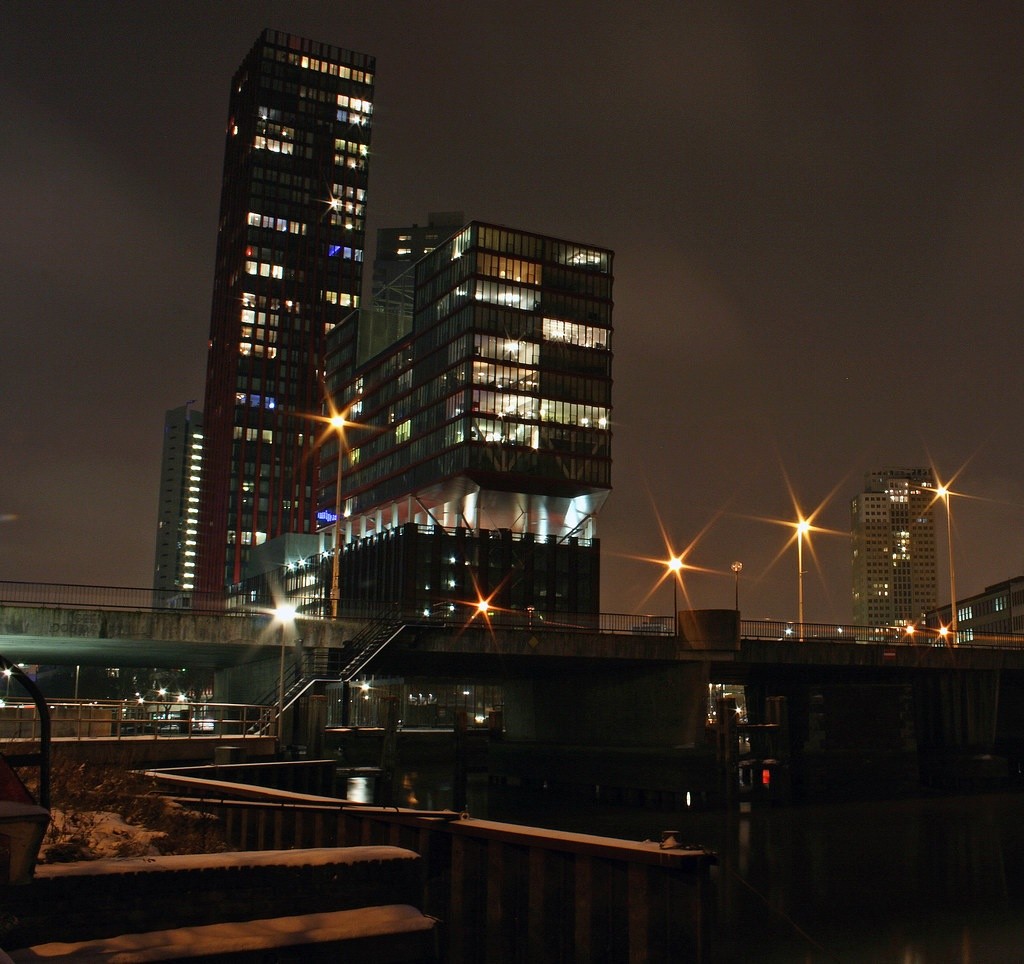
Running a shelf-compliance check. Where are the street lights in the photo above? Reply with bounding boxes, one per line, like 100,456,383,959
668,558,682,634
797,521,808,639
329,414,345,616
730,563,744,609
275,608,296,735
936,488,957,645
